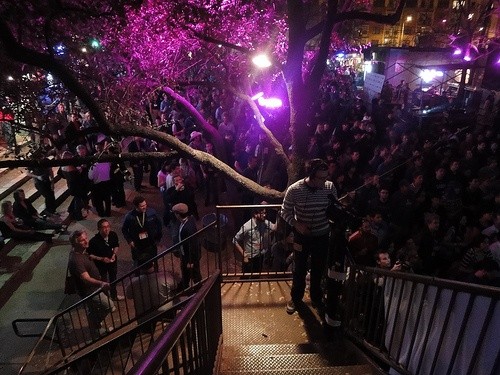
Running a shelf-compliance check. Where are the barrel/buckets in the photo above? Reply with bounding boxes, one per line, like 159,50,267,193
202,213,228,253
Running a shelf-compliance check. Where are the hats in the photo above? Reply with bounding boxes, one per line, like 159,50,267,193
170,203,189,213
305,158,327,175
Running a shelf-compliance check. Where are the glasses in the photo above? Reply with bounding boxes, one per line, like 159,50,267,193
311,174,329,180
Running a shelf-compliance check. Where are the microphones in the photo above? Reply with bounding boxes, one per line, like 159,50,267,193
326,205,358,230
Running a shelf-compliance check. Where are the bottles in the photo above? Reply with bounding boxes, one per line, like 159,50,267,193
330,262,341,272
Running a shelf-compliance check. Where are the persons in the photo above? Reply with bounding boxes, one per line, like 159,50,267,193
0,62,500,339
280,158,342,314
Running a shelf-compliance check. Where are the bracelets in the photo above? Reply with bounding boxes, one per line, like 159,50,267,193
101,281,104,285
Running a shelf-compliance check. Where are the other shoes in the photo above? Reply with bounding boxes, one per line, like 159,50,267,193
174,285,189,292
59,224,67,233
88,328,107,336
50,232,60,242
111,295,125,301
287,299,302,313
110,306,116,312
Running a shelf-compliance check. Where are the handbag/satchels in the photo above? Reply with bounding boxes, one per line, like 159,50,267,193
64,276,77,295
